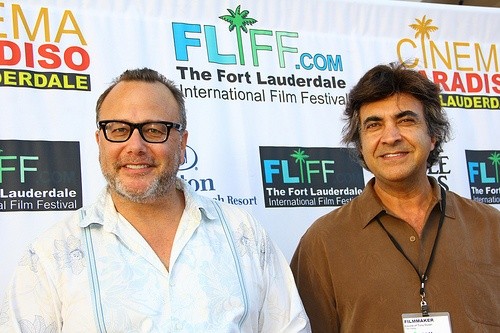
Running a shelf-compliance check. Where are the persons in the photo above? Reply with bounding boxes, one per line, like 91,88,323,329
290,64,500,333
1,67,311,333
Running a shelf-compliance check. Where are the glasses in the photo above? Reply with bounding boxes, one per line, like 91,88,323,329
96,118,183,143
